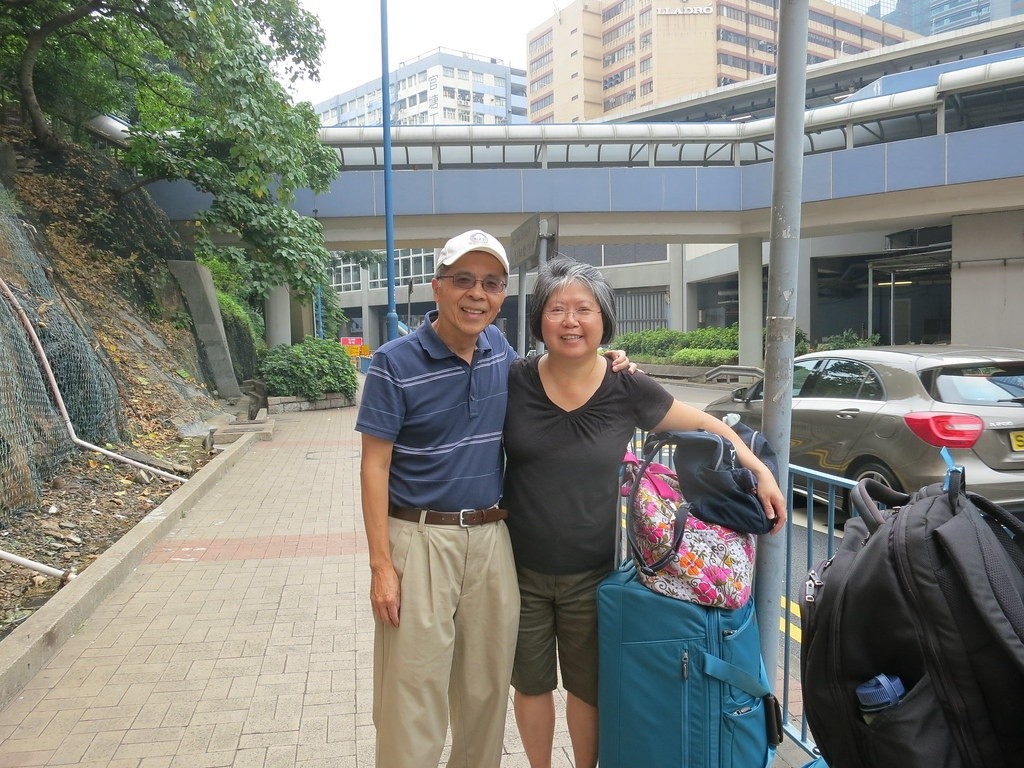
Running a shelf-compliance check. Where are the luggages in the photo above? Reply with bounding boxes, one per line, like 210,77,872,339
597,465,784,768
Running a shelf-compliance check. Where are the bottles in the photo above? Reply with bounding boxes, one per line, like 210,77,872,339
855,673,906,725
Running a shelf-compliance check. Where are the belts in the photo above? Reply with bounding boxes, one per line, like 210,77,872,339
388,506,506,527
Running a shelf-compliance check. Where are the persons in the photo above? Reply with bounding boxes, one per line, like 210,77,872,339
354,230,643,767
504,260,785,767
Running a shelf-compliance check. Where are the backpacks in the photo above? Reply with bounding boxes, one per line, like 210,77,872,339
798,465,1024,768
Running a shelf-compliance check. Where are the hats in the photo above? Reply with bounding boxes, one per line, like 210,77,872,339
437,230,509,276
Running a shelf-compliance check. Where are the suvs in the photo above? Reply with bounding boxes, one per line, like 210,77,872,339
696,346,1024,523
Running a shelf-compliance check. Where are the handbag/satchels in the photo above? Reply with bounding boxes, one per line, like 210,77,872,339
619,452,756,608
625,420,779,576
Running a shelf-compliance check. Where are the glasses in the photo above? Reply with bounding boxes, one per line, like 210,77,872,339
543,307,603,322
436,275,507,294
